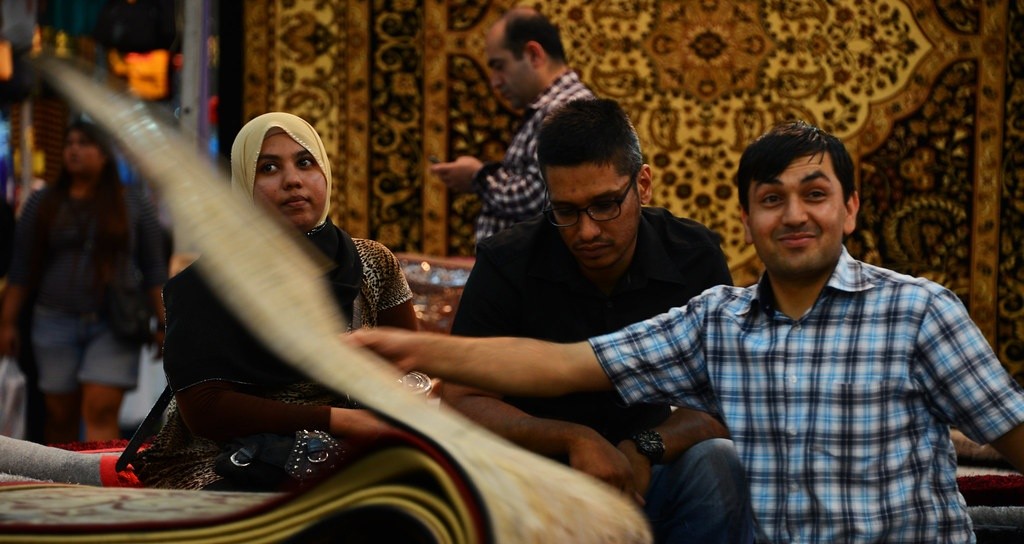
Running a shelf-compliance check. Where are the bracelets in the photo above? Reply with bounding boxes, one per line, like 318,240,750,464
158,322,166,332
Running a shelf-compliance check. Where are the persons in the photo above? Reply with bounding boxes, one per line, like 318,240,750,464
0,119,167,442
334,4,1024,544
162,112,423,493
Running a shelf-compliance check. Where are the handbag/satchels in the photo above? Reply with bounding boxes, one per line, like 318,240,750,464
215,430,352,494
0,354,27,441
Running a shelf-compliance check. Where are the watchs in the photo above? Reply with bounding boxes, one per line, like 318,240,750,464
627,426,666,467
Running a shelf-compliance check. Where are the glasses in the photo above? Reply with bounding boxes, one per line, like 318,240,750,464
541,169,635,227
